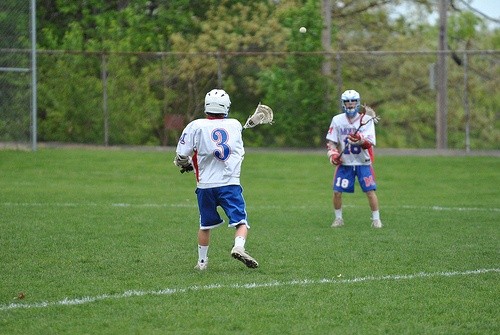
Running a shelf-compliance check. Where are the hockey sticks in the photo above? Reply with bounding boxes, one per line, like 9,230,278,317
335,104,377,160
181,103,274,173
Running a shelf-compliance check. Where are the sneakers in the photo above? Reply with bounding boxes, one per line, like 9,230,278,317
231,245,258,268
331,218,344,227
371,219,381,228
194,260,208,270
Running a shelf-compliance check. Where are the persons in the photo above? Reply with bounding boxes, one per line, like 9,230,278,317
326,89,383,228
176,88,259,270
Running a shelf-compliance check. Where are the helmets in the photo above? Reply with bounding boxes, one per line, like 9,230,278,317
204,89,231,117
341,90,361,117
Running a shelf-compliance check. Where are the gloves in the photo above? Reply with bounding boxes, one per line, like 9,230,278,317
176,154,194,174
327,149,343,165
347,132,371,149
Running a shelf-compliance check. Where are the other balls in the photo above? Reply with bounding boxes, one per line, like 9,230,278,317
300,27,307,33
